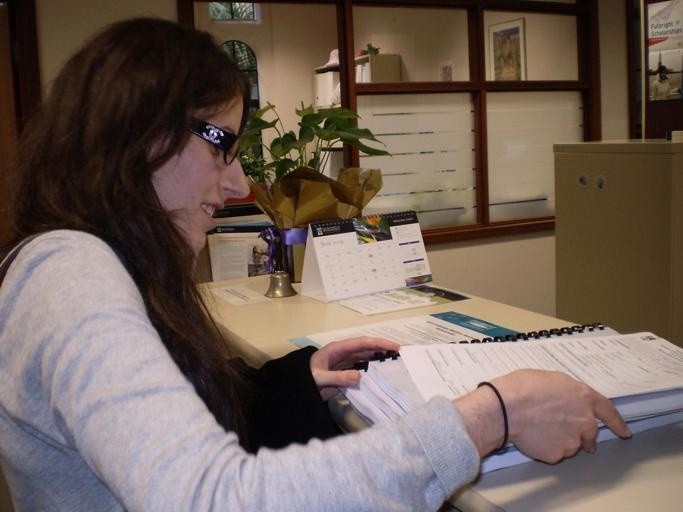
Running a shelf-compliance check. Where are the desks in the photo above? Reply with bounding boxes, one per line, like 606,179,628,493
188,275,682,511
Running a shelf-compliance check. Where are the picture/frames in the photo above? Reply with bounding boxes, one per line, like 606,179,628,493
487,18,527,82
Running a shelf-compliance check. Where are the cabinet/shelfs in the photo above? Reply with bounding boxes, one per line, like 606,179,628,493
552,137,683,350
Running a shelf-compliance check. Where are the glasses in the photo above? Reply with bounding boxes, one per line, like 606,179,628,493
181,111,244,166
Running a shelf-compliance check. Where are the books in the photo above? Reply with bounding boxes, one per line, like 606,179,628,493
338,324,683,474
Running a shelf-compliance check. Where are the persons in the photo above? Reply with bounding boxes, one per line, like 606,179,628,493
1,13,634,511
650,66,672,100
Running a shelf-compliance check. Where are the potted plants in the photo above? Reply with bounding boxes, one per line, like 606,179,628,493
234,99,392,284
